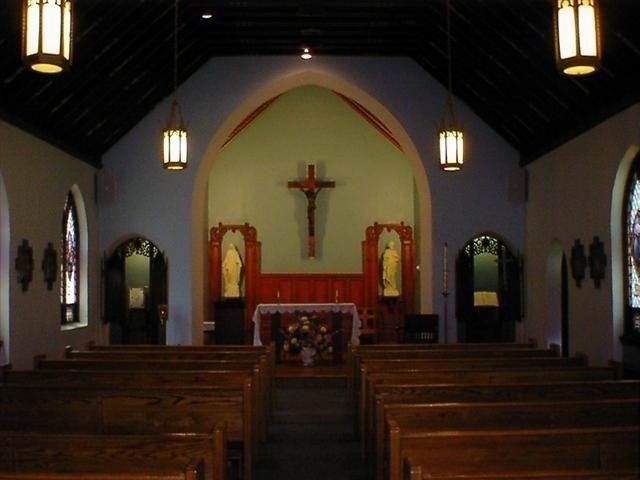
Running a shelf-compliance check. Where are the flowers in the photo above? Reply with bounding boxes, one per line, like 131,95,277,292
277,313,338,350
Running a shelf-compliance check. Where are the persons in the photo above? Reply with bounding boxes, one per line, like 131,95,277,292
381,240,401,297
222,243,245,299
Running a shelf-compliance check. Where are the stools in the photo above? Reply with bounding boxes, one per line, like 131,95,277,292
0,338,272,478
346,335,636,479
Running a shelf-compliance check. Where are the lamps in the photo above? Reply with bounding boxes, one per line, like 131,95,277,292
159,2,189,169
438,2,464,172
551,1,604,80
18,2,75,75
